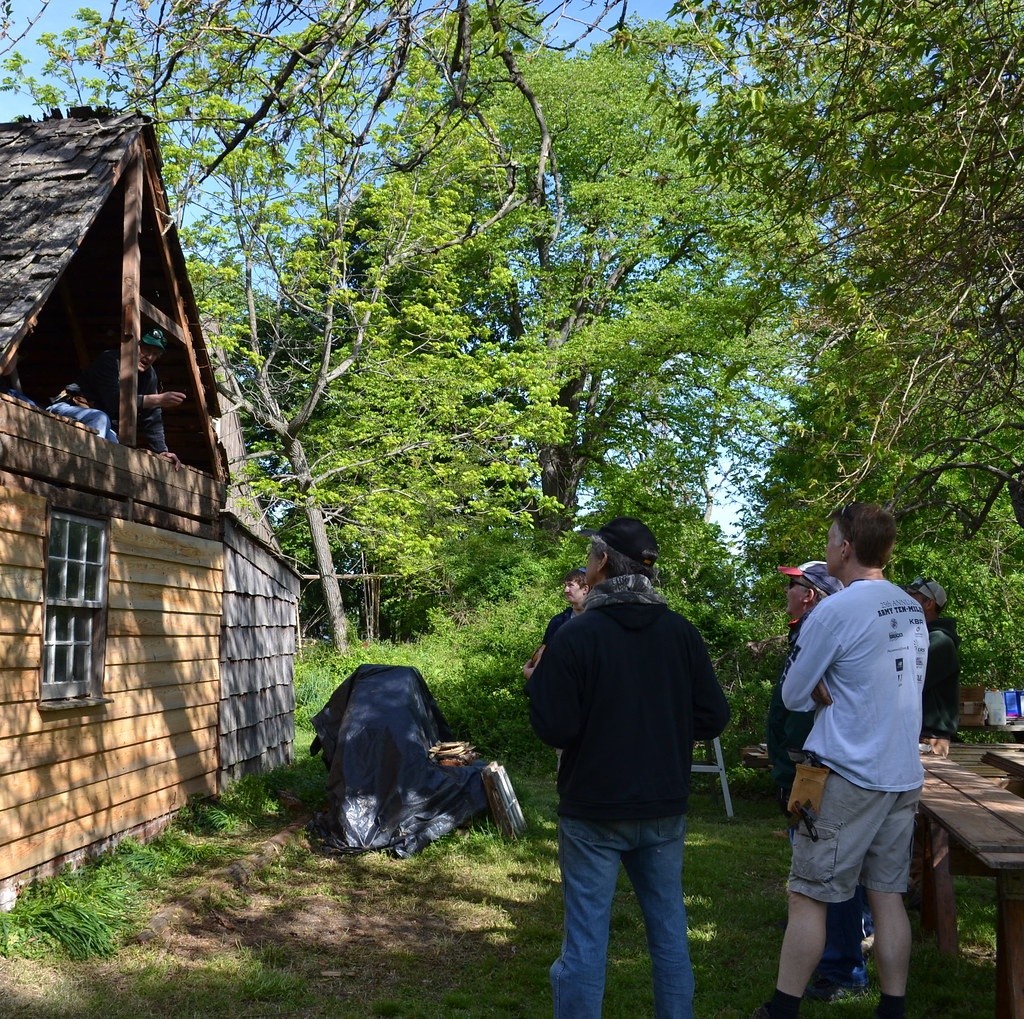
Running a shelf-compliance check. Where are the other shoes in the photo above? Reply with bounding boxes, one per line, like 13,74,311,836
752,1001,771,1019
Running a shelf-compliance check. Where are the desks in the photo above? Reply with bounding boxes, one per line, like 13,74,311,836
981,753,1024,798
959,715,1024,742
918,752,1024,1019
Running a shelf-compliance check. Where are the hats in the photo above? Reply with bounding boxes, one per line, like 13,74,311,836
591,517,660,581
141,319,168,353
778,560,844,596
899,577,948,610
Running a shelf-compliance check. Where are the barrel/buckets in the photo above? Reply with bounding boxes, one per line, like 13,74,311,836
986,686,1007,725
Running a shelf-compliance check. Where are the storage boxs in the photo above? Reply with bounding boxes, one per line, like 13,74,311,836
959,685,985,725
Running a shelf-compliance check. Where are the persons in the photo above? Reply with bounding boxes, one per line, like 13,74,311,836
897,576,960,743
524,517,730,1018
751,503,929,1019
542,567,589,647
45,325,187,471
767,559,868,999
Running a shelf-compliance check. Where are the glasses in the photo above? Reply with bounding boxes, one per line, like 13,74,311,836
150,329,167,348
789,577,817,598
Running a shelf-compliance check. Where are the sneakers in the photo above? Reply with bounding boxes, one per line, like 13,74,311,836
801,979,869,1003
